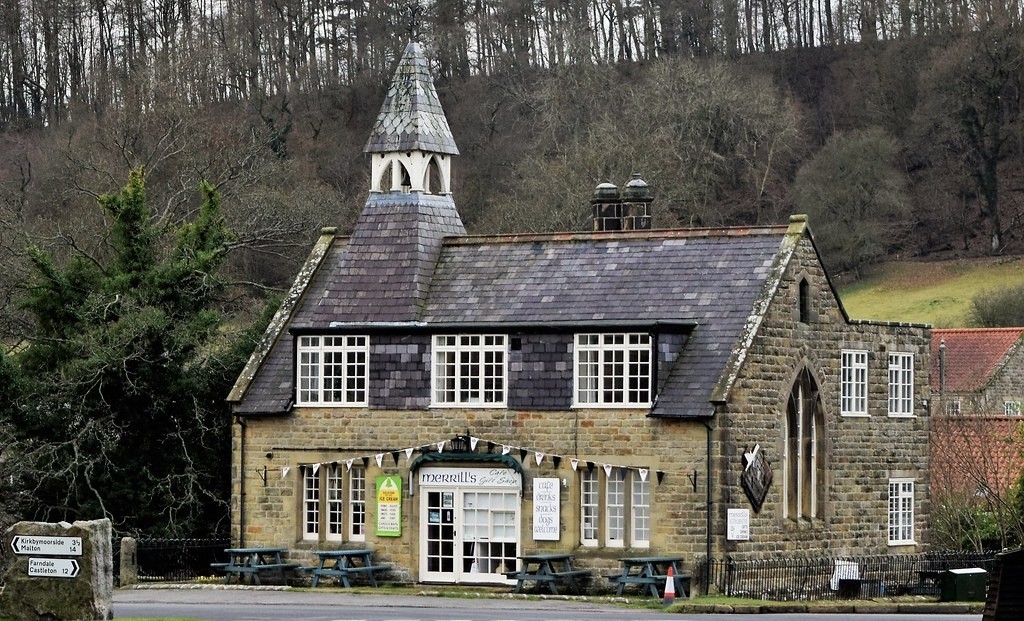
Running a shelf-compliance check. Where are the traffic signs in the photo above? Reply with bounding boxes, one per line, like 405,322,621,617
10,535,82,556
28,558,80,578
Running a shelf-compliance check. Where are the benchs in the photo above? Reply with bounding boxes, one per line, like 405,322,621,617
300,549,389,588
211,548,300,586
501,554,590,596
602,557,692,597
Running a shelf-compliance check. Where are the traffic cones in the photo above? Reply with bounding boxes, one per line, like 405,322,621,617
663,566,676,606
